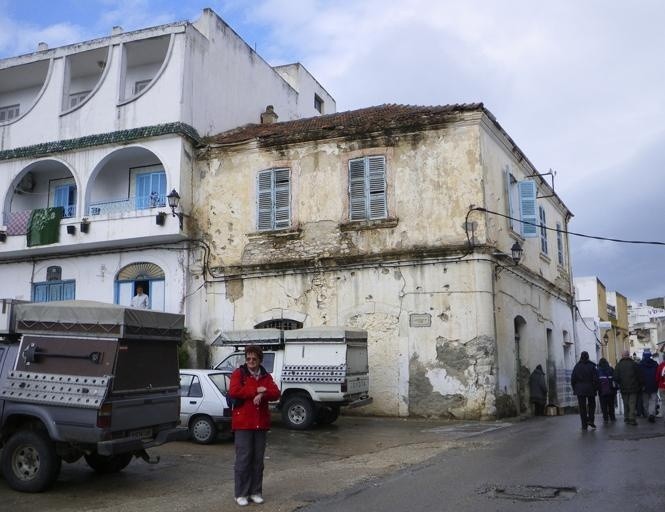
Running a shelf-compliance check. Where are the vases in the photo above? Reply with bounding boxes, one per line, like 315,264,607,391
0,234,6,242
67,225,75,234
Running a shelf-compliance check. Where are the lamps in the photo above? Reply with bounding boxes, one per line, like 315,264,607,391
602,332,609,346
166,187,183,231
494,240,523,282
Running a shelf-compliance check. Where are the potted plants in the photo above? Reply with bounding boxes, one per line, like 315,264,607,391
80,218,91,233
156,212,167,226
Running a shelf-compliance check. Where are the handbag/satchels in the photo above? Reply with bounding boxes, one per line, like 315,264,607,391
226,393,244,409
609,379,619,390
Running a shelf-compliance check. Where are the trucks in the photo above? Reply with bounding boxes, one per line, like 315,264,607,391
0,292,191,497
206,326,374,433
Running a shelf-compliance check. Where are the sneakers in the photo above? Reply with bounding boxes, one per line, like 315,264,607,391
583,420,596,432
250,495,264,504
604,413,662,425
235,496,248,506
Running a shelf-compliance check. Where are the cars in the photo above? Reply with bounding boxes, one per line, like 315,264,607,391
166,361,237,446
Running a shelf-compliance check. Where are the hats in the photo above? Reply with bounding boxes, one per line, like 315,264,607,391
643,352,651,357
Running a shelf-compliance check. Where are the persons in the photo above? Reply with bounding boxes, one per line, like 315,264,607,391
570,351,601,429
229,344,281,506
527,364,548,416
596,348,665,425
131,283,149,309
